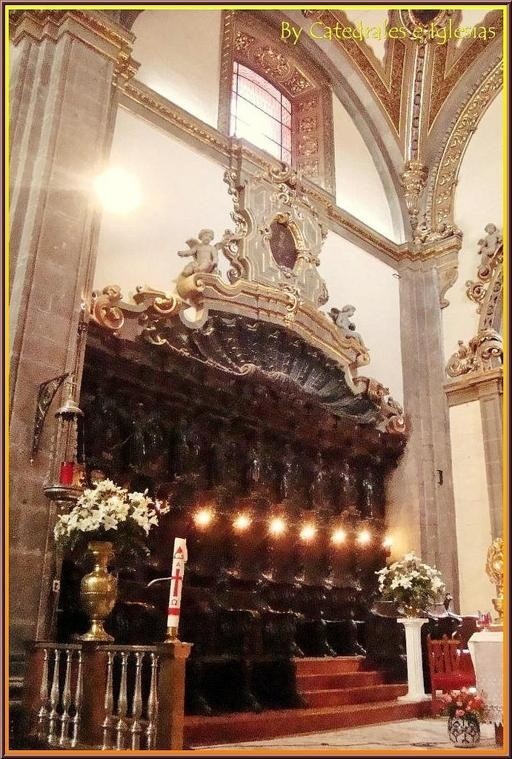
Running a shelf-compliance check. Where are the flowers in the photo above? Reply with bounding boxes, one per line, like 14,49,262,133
372,550,449,622
50,475,175,550
437,683,496,726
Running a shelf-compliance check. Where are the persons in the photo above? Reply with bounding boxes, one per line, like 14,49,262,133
478,222,499,268
176,226,221,275
335,305,369,353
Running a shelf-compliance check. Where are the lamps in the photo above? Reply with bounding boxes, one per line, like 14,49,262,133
27,368,86,468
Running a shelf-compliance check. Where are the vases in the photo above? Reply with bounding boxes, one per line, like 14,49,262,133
444,712,482,750
70,534,126,646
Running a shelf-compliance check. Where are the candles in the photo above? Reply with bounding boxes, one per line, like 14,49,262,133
160,525,193,653
54,450,81,494
